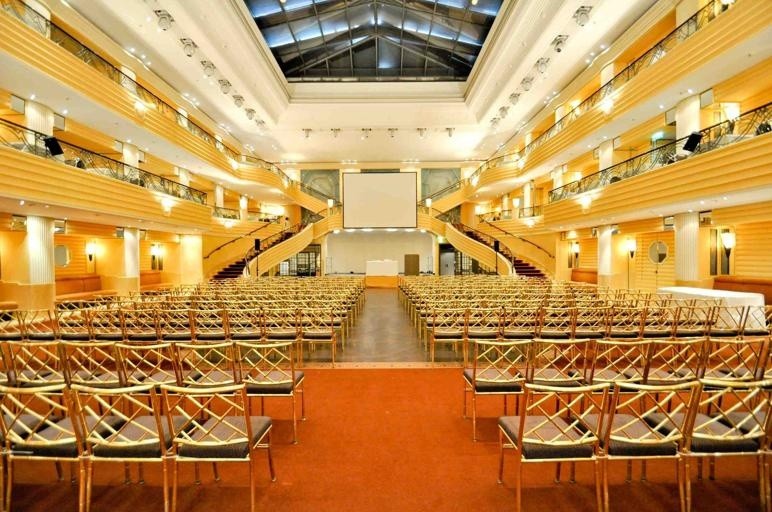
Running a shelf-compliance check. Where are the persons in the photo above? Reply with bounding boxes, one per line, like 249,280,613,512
282,217,289,240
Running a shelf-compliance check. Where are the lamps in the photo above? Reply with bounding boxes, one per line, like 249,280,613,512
500,6,592,118
154,9,265,127
